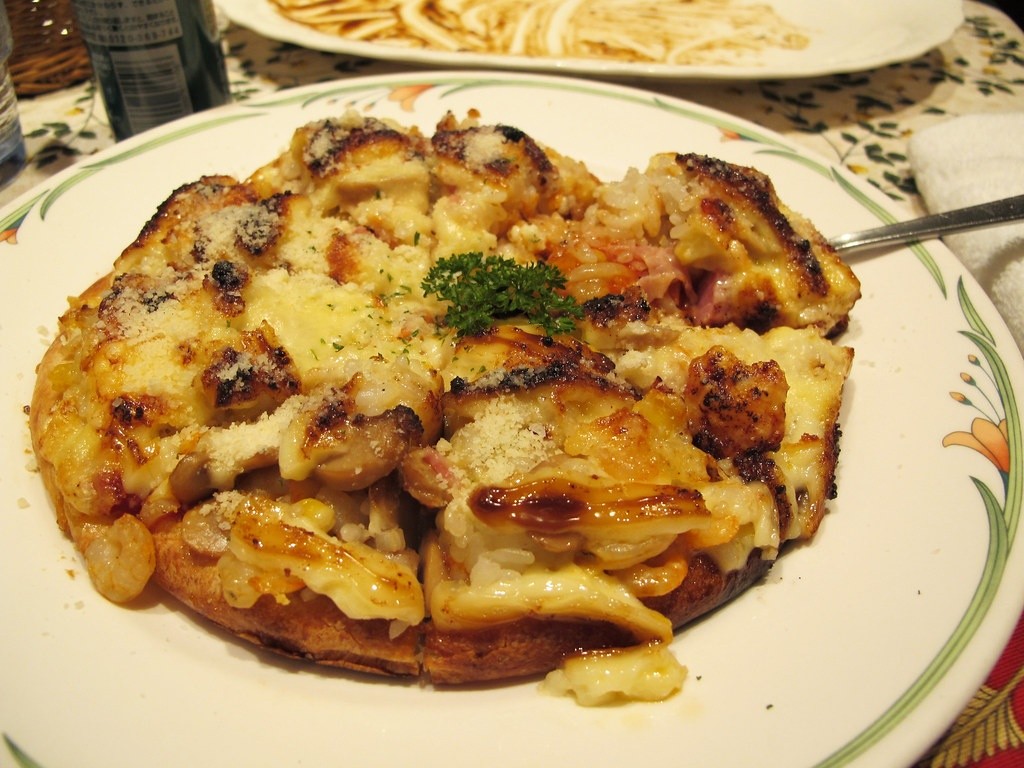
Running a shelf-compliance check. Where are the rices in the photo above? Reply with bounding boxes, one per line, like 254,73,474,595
340,150,692,583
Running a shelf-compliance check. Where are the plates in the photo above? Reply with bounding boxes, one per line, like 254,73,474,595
215,0,966,82
0,73,1024,768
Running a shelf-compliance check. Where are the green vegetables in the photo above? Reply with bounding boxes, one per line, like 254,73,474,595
420,251,586,344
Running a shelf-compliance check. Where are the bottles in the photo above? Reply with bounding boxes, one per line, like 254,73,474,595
67,0,232,148
0,0,29,190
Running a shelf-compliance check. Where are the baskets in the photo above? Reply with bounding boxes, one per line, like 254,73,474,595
3,0,94,95
